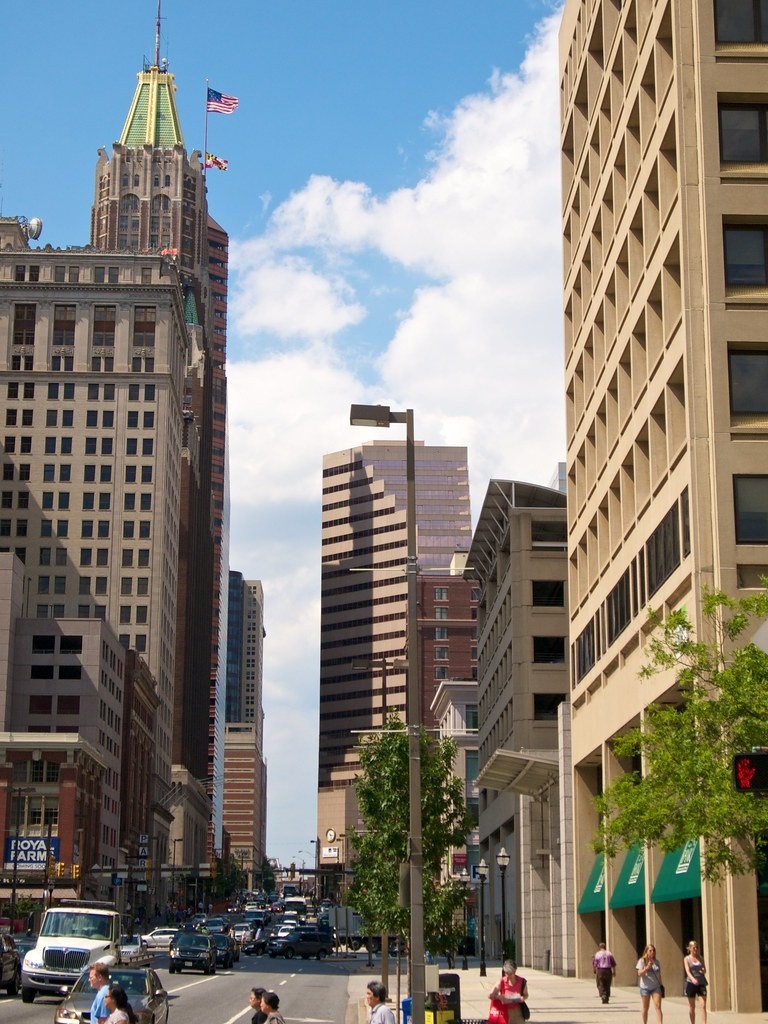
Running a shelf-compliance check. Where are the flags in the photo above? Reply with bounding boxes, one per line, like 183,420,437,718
206,152,228,171
207,88,239,113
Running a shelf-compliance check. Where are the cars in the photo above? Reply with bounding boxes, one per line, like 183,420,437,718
54,963,169,1024
168,883,382,976
120,934,144,963
141,927,180,949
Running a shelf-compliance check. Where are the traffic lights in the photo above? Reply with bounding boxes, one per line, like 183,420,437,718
58,861,65,877
730,751,768,795
73,864,79,880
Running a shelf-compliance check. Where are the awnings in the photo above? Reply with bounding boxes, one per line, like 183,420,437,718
609,839,645,909
577,854,605,913
0,885,44,898
53,887,77,898
650,832,701,903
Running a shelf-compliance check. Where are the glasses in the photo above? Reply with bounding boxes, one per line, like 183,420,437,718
105,994,112,998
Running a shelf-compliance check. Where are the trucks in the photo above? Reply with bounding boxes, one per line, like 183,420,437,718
20,898,136,1003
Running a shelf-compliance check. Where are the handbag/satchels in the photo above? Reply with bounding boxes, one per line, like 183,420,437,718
660,984,666,998
520,978,530,1020
487,979,508,1024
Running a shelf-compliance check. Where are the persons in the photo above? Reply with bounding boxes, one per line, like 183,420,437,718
167,901,213,923
220,926,275,947
88,964,111,1024
104,984,138,1024
489,960,529,1024
178,922,211,933
367,981,395,1024
227,887,272,916
593,943,616,1003
126,901,159,927
250,988,285,1024
684,941,708,1024
636,945,663,1024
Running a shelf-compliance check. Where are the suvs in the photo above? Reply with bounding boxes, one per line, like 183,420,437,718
0,933,23,997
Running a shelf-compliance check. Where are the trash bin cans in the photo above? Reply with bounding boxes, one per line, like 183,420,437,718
431,973,461,1024
402,997,413,1024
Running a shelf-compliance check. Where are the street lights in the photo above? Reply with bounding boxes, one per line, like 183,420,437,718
3,785,38,935
348,402,427,1024
350,656,411,733
459,867,470,971
336,838,344,894
339,834,347,890
309,840,320,902
172,838,184,921
496,846,511,978
48,881,54,908
476,858,489,976
297,850,317,898
292,855,305,894
